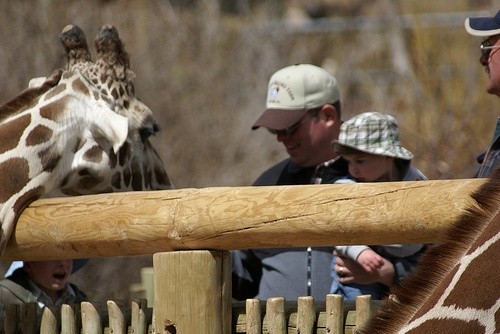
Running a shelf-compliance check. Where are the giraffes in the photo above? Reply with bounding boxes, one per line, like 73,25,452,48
0,16,176,260
353,151,499,334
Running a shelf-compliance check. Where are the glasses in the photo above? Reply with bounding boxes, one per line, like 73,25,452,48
480,40,500,50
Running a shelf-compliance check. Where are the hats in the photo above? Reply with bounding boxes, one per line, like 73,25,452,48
465,10,500,37
251,63,340,130
331,111,415,160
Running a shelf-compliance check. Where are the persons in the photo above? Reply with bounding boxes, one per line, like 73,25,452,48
1,252,89,320
227,62,435,299
330,112,432,300
465,4,500,179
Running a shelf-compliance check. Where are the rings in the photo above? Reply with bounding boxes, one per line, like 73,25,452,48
339,267,343,275
